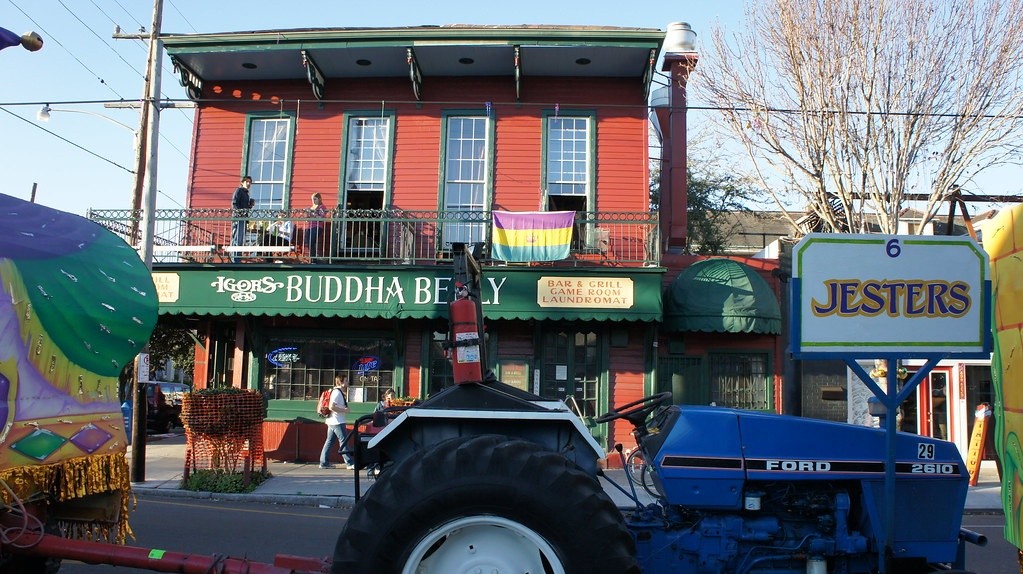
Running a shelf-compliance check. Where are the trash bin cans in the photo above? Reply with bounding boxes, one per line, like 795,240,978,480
121,399,133,445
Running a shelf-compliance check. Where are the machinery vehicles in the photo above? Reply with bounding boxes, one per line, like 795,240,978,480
0,190,991,573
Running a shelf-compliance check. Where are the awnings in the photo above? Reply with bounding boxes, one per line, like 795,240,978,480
151,259,782,335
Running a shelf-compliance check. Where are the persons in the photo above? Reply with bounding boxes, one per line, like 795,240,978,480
319,375,354,470
256,213,293,263
366,388,396,476
230,176,255,263
307,192,327,265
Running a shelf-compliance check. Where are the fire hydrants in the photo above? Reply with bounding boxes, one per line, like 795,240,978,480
120,402,132,446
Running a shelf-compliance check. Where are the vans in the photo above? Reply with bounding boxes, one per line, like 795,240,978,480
147,383,190,433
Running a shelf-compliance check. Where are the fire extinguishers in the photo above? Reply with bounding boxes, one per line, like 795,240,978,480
440,273,482,384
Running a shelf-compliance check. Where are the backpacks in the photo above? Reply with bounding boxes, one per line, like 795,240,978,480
317,388,348,418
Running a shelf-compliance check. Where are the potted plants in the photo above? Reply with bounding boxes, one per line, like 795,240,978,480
388,396,419,415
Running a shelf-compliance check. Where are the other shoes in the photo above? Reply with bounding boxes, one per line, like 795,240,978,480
347,464,355,470
319,465,336,468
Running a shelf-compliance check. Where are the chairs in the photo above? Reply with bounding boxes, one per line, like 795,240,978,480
245,224,297,263
